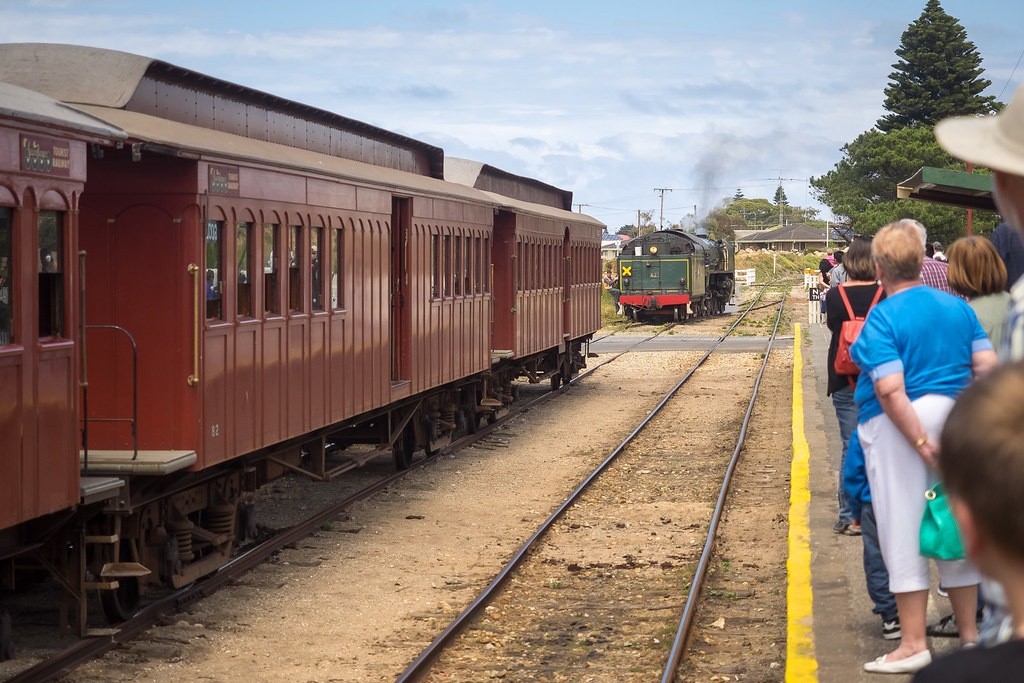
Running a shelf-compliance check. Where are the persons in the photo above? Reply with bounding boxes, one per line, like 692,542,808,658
820,225,1012,642
912,359,1024,683
855,222,999,674
935,88,1023,235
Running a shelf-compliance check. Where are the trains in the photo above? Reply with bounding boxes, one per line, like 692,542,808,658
0,41,608,641
615,229,737,324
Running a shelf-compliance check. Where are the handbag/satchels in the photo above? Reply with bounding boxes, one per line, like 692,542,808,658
918,457,969,561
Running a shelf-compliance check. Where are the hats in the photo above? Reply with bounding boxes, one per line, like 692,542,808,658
933,241,942,248
934,83,1024,177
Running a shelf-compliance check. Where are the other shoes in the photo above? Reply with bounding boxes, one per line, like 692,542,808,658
834,519,862,536
926,614,981,637
863,649,933,673
881,616,903,640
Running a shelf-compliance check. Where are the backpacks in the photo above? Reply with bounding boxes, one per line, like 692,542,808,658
834,283,885,374
